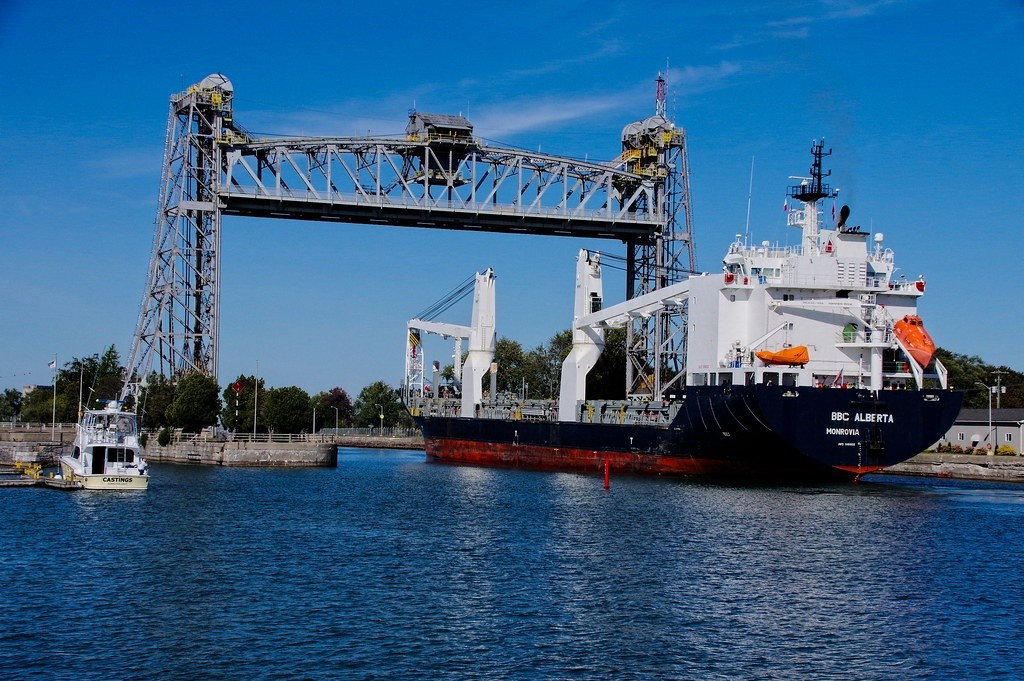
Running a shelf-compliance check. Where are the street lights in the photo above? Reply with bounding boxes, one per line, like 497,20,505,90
253,360,259,443
50,351,58,442
331,406,338,435
314,406,316,443
974,382,992,450
375,404,383,436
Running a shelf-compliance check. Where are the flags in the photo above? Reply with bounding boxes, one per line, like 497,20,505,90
783,198,787,213
48,360,56,369
834,369,842,383
831,203,835,221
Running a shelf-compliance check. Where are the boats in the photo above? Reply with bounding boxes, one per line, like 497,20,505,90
46,477,82,491
60,395,149,490
399,137,962,478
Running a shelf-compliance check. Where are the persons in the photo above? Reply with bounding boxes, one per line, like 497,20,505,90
817,380,866,389
